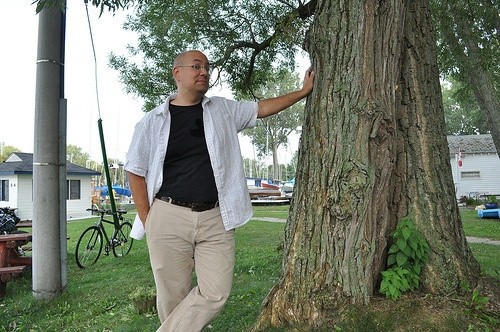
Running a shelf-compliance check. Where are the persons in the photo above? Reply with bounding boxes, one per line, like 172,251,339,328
124,51,315,332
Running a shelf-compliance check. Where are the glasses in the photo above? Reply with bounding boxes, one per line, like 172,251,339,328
178,64,212,74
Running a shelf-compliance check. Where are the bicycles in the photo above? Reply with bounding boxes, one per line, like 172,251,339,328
75,208,134,269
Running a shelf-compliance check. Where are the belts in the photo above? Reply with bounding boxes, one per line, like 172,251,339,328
155,194,219,212
